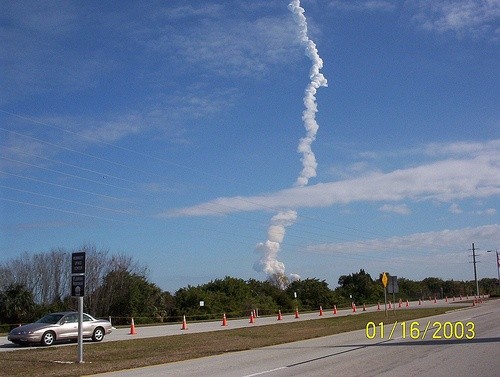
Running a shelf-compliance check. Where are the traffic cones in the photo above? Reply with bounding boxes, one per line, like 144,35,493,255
128,319,137,335
434,297,437,303
249,309,256,323
333,305,337,314
399,299,409,308
319,306,323,316
377,302,381,310
221,314,228,326
419,299,422,305
277,310,283,320
388,302,392,309
181,316,188,330
295,308,299,318
446,294,484,307
352,302,357,312
429,297,431,301
362,304,366,311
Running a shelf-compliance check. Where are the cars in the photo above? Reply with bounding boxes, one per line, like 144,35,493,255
8,312,113,346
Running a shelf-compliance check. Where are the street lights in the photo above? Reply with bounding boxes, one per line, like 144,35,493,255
487,250,500,283
